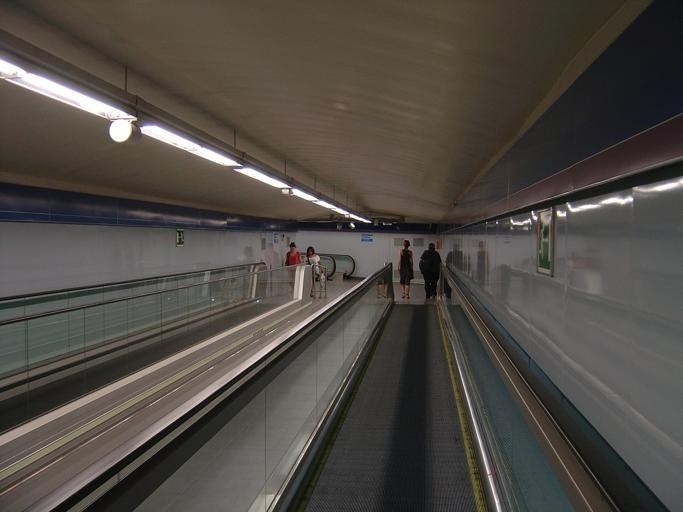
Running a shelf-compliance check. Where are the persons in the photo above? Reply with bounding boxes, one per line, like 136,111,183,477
418,242,441,301
284,242,302,299
445,243,465,271
396,239,414,299
304,246,323,297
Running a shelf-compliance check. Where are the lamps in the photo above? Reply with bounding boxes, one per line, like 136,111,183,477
345,213,372,223
108,119,143,143
233,164,293,189
312,198,349,215
290,186,319,201
2,51,138,124
140,123,244,169
